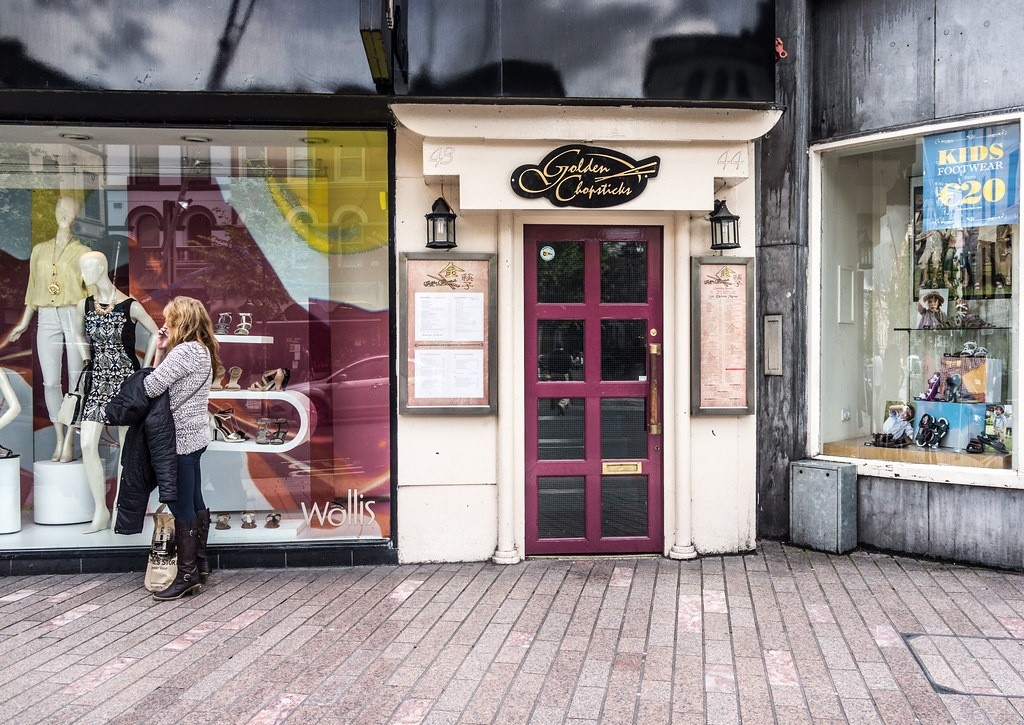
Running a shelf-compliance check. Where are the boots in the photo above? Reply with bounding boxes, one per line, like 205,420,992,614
194,508,212,584
152,519,202,601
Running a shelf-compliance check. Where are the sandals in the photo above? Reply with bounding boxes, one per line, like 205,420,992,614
921,372,940,400
923,418,949,447
0,444,21,458
974,347,988,358
916,414,936,446
960,340,977,357
241,512,257,529
1005,276,1011,285
944,353,951,357
966,438,984,453
265,512,281,529
951,351,962,357
921,280,930,288
215,513,231,531
995,281,1002,289
932,281,938,288
943,374,962,403
975,283,981,290
977,430,1009,453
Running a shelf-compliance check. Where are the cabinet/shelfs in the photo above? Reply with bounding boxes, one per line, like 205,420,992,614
203,333,317,454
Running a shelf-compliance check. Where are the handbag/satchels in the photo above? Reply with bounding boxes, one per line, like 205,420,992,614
57,364,93,427
144,503,178,591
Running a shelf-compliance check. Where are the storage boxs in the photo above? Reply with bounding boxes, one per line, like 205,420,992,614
938,356,1002,403
911,401,985,449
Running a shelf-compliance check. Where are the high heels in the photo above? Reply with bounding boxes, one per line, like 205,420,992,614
268,368,290,392
210,365,226,391
216,403,251,440
248,368,284,391
270,416,289,445
225,366,242,391
214,312,233,335
233,311,253,335
256,418,273,444
210,414,245,443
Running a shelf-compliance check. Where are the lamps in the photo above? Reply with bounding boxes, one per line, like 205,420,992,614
425,198,457,250
708,198,741,250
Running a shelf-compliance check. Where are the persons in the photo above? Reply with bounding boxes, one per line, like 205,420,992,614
0,369,22,430
0,195,93,464
546,339,581,416
143,296,222,600
74,251,163,534
880,202,1012,448
